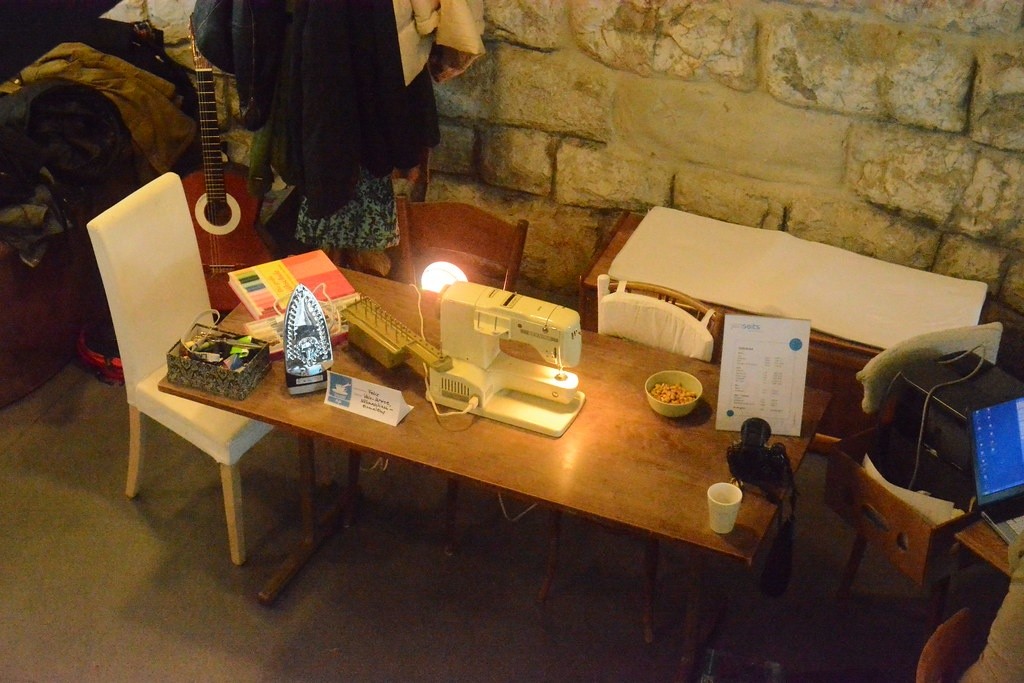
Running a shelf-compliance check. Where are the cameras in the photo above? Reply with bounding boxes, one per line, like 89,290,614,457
726,417,790,490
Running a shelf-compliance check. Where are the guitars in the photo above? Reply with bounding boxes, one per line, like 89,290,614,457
175,14,273,312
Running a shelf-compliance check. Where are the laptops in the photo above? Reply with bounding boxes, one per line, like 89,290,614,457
966,390,1024,546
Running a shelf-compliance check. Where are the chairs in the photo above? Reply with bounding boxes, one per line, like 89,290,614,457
534,272,723,648
85,171,322,564
343,191,533,549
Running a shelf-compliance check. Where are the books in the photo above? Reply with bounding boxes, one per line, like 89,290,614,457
225,249,356,322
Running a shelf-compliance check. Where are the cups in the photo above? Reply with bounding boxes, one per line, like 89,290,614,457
707,482,743,534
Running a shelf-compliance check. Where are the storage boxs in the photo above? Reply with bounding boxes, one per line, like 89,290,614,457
164,321,273,403
0,182,116,411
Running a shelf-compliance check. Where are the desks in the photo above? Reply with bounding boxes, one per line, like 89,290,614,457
157,255,838,683
574,205,994,457
931,514,1014,637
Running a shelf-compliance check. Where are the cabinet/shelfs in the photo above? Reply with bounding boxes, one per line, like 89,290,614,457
825,419,979,650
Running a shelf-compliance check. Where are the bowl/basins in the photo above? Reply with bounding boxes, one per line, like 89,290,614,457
644,370,703,418
